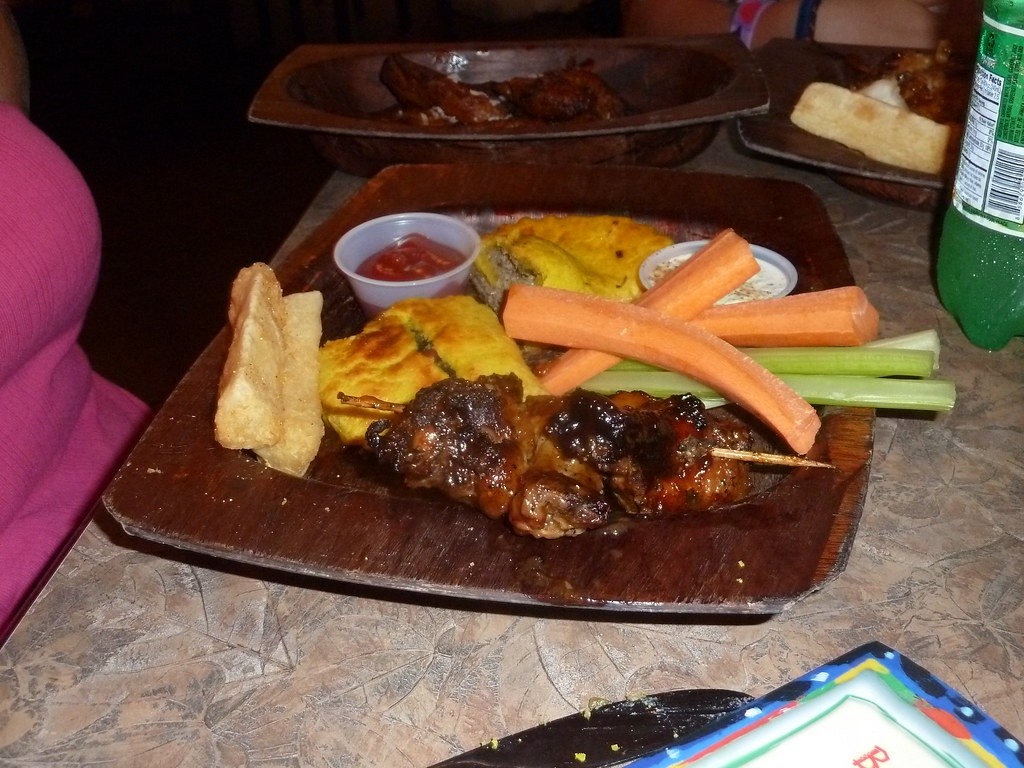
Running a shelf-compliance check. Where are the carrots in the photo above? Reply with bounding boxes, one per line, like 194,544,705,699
501,226,880,456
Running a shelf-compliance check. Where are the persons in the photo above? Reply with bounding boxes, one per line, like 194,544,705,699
621,0,982,49
0,0,155,633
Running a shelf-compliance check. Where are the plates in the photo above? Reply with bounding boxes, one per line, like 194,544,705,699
246,32,771,167
426,688,755,768
739,37,974,207
99,161,875,619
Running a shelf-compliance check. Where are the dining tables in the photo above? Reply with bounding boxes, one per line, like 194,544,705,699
0,124,1024,768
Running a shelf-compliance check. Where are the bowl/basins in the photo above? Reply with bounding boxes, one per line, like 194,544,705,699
335,213,480,318
639,239,797,305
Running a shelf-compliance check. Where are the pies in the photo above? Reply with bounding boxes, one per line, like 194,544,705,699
313,292,543,445
467,210,672,309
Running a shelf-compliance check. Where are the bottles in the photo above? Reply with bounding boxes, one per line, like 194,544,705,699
935,0,1024,350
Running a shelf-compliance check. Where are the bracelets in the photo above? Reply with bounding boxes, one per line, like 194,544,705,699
730,0,773,49
794,0,821,41
